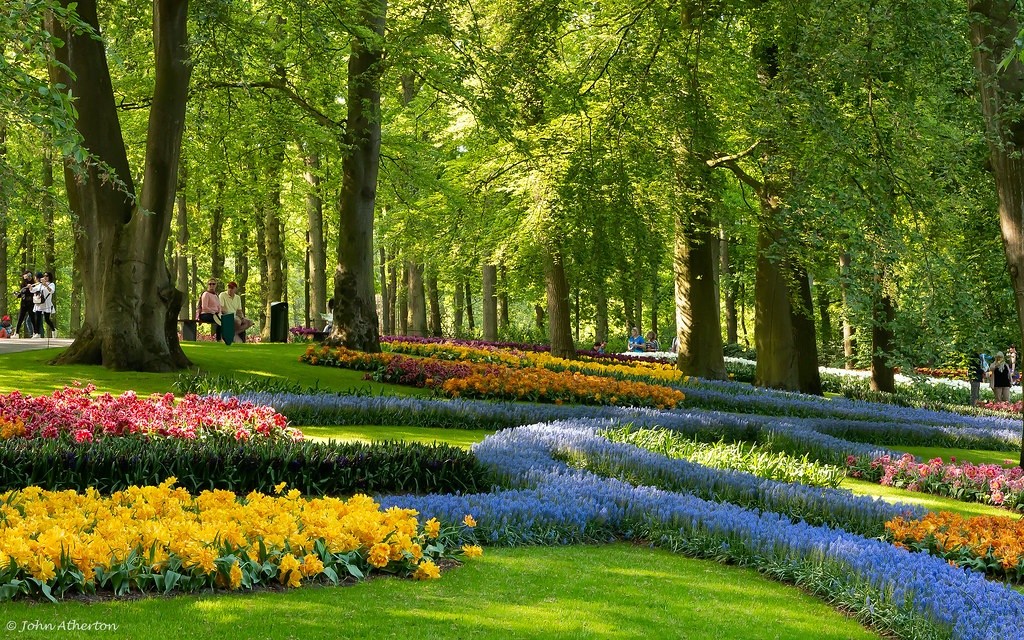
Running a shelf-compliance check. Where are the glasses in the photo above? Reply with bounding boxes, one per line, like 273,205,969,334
42,275,49,277
208,283,216,285
227,286,234,289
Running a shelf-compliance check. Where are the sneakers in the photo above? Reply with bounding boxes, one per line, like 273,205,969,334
31,333,41,340
10,331,19,339
52,328,59,339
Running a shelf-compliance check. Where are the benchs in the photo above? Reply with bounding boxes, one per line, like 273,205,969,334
308,331,329,341
176,319,246,343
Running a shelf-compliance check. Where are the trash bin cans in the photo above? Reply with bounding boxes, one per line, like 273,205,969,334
269,301,289,343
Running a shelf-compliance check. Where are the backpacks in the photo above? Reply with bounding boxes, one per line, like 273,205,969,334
33,282,52,304
198,291,205,309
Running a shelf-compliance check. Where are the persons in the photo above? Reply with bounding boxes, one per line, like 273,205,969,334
978,345,1023,386
671,336,677,354
590,342,601,353
322,298,334,333
627,327,646,353
968,350,990,407
197,278,225,343
989,351,1013,402
0,272,58,338
598,340,608,354
645,331,659,352
218,281,254,343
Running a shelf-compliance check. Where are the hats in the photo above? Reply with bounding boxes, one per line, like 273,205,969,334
2,315,11,321
227,282,237,288
